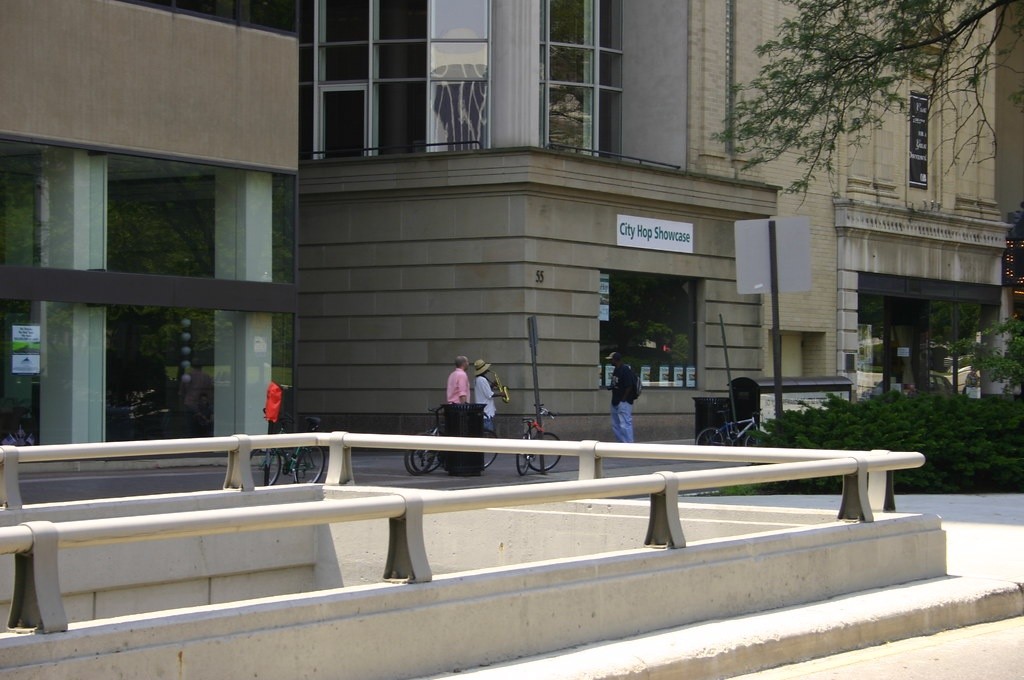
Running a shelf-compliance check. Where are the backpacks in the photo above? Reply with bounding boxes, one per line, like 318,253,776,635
620,365,642,396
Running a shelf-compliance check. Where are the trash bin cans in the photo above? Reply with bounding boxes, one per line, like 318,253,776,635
693,396,732,447
441,402,487,476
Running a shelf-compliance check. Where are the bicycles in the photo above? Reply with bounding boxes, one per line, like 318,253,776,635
515,402,564,477
696,407,770,452
249,406,325,484
405,402,500,476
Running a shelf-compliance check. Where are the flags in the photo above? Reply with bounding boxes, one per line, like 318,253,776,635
265,381,283,423
26,433,36,446
1,435,16,445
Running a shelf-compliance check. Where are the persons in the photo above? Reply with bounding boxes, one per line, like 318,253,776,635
472,359,507,433
447,356,470,408
142,354,220,436
599,351,635,442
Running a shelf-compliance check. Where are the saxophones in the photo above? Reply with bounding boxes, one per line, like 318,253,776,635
487,369,510,404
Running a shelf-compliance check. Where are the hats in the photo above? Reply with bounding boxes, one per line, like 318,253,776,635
474,360,491,375
605,352,622,360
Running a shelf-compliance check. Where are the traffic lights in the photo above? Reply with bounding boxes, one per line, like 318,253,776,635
1000,239,1024,285
